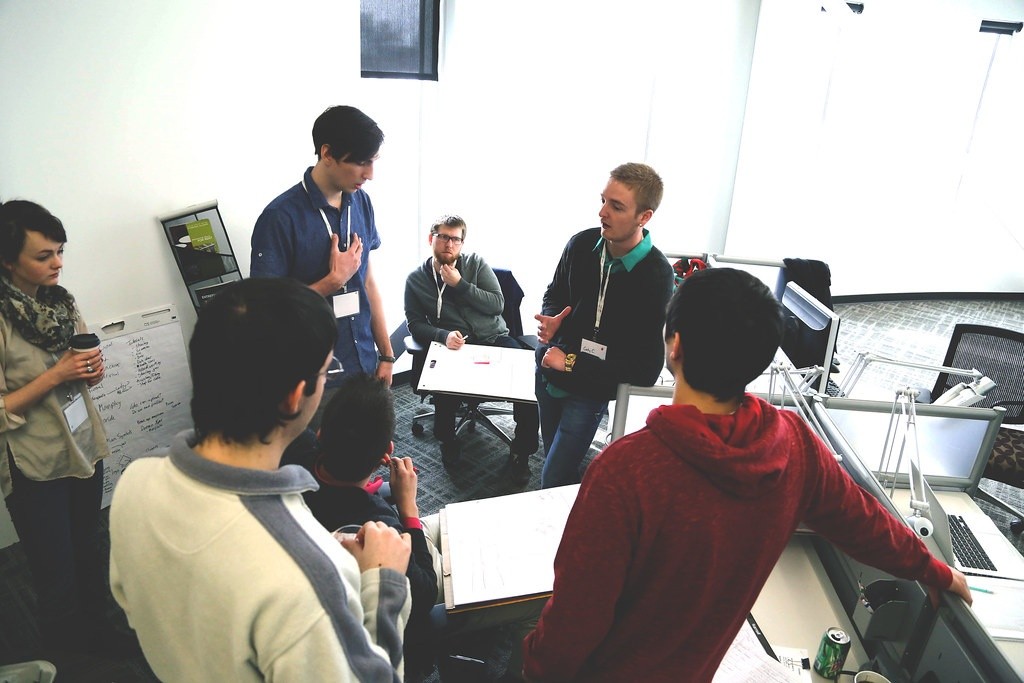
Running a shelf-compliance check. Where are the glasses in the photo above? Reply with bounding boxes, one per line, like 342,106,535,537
290,357,344,387
433,233,463,245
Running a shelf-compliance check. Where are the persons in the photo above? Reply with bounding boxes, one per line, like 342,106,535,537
404,214,540,486
251,106,396,435
0,200,143,659
523,268,972,683
110,276,412,683
280,372,549,683
534,163,674,489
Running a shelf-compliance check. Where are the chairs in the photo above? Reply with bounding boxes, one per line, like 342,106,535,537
928,323,1023,422
405,269,523,455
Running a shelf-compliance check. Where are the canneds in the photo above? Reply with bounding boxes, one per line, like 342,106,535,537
813,627,851,679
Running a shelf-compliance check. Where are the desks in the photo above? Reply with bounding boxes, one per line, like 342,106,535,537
608,256,1024,683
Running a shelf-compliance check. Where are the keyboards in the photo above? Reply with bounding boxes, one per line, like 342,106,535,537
825,377,845,398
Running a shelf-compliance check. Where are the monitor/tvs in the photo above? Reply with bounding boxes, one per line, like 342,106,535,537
781,280,841,394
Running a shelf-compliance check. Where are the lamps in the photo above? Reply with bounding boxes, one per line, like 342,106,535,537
877,391,933,540
838,352,996,406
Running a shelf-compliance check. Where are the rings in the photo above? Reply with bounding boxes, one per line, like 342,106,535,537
88,367,95,373
87,360,91,366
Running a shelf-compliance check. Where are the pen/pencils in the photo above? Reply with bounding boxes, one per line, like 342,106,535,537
462,335,468,339
387,460,419,472
858,580,874,613
969,586,994,594
474,361,489,364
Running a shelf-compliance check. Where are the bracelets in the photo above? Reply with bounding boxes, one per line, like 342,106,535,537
379,356,395,363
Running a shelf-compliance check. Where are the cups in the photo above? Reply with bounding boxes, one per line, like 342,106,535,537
69,333,101,356
854,670,891,683
331,524,363,546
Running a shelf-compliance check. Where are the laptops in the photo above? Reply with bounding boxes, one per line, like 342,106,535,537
909,458,1024,580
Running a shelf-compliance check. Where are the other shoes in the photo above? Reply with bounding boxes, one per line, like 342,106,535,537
509,427,539,454
37,614,141,659
433,407,455,443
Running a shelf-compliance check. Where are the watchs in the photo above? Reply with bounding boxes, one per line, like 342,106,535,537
565,354,576,372
